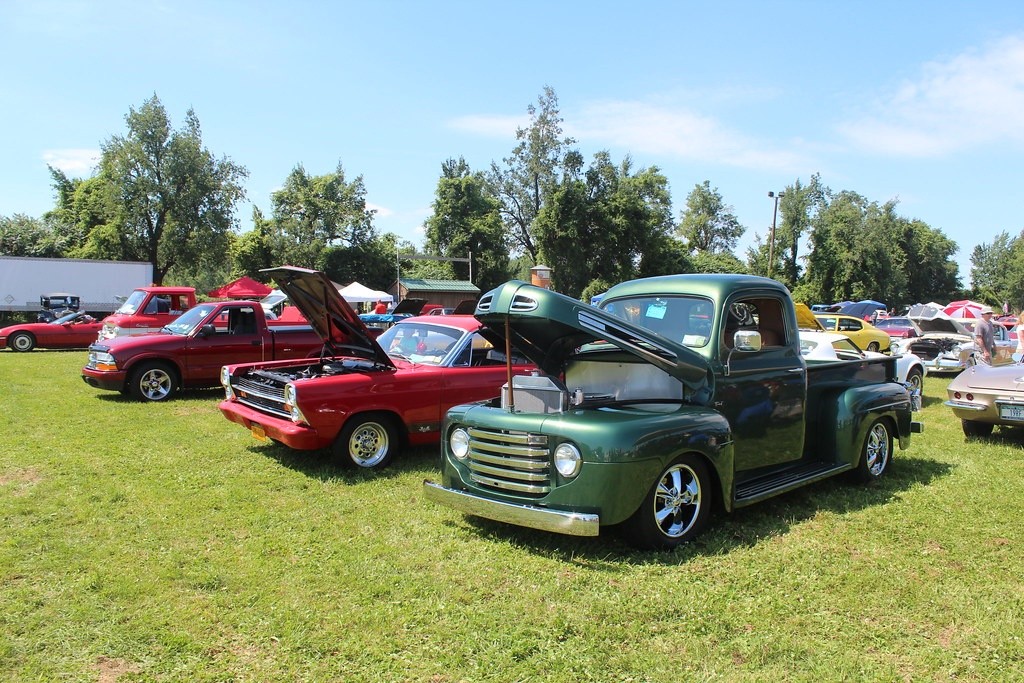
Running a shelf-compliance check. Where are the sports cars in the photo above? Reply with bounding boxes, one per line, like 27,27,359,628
0,312,103,352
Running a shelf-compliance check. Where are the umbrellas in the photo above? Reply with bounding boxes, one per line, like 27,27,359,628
943,300,984,332
924,302,945,310
858,299,887,309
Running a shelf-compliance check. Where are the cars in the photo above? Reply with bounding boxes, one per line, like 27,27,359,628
421,273,925,552
359,297,480,331
38,295,81,323
810,300,1020,340
944,354,1024,439
890,304,1020,373
215,265,561,469
877,315,915,343
793,302,891,354
799,332,928,402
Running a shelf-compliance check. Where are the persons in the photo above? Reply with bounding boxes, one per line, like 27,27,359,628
973,306,994,365
1015,310,1024,363
399,328,418,350
375,300,387,331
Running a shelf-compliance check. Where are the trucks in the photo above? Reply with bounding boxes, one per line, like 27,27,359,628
94,284,311,343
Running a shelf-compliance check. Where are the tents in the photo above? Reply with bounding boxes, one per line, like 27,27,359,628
337,281,393,313
208,275,283,314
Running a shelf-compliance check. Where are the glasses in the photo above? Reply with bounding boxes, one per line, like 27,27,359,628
986,312,992,315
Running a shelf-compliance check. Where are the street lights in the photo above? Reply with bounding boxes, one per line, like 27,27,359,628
767,191,786,281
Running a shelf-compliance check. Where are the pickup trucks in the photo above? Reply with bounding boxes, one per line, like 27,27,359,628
80,301,386,404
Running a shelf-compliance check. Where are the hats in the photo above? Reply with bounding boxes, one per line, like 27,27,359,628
980,306,995,314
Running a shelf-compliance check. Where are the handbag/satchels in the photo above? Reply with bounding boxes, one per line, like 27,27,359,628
1011,353,1024,363
